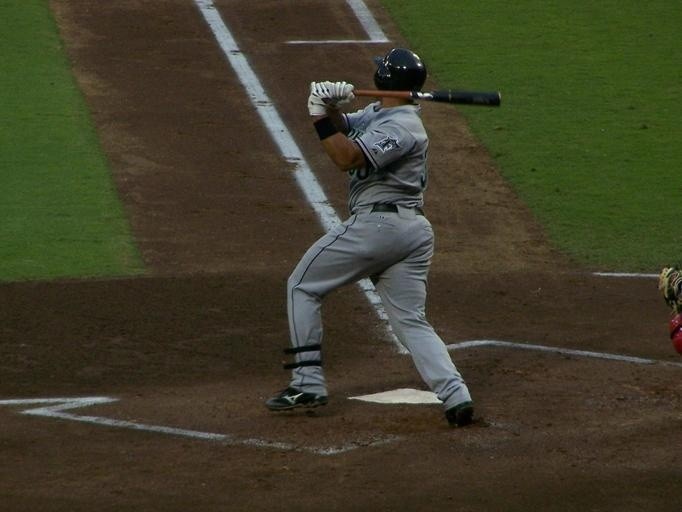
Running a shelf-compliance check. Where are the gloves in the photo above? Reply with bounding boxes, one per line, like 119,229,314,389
308,81,354,115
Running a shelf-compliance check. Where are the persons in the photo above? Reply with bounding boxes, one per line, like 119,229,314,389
266,47,476,426
659,266,682,356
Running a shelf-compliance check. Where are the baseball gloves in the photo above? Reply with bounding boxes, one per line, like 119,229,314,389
659,267,682,314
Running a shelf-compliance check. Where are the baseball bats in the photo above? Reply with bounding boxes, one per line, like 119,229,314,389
352,89,502,106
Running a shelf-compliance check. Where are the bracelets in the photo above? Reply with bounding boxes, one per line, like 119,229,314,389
312,117,337,141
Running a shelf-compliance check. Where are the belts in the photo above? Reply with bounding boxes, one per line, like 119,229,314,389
370,202,423,214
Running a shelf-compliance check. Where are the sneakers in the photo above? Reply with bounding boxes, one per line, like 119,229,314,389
266,387,328,411
445,402,473,426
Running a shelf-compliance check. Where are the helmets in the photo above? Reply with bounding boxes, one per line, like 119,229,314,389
372,46,425,92
